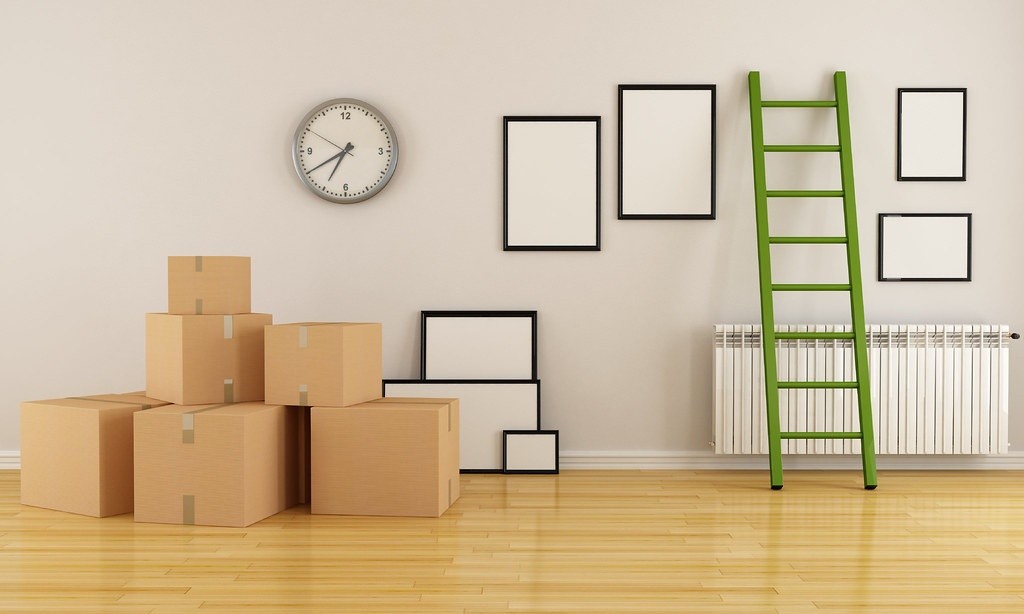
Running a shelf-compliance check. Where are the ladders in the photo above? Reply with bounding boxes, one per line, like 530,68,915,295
747,69,877,489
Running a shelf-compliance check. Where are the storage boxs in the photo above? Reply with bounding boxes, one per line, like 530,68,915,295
22,255,462,527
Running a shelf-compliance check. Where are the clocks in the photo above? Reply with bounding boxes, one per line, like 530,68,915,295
291,95,400,206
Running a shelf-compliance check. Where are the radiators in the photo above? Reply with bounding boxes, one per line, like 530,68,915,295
713,323,1013,458
